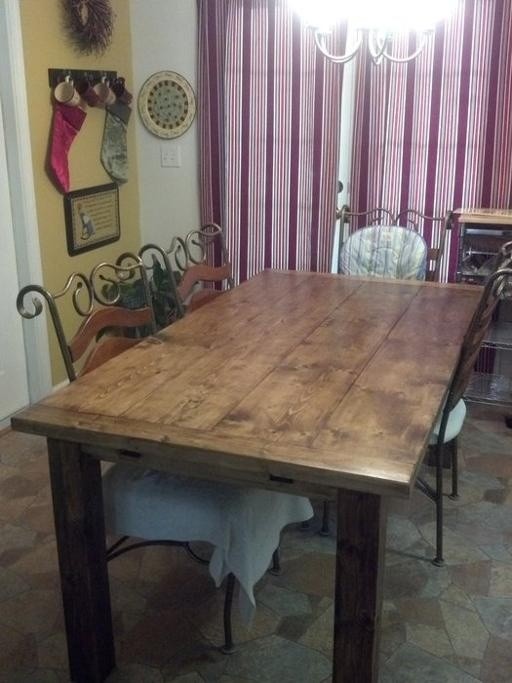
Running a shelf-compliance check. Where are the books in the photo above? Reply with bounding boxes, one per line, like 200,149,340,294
466,226,505,236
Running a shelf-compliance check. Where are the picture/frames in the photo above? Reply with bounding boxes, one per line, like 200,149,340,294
63,182,120,256
137,71,196,139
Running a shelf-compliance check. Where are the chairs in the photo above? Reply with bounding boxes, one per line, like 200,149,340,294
17,251,316,654
335,205,451,282
320,241,512,565
137,223,234,317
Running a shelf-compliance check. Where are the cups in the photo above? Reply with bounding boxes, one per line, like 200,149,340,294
53,75,133,108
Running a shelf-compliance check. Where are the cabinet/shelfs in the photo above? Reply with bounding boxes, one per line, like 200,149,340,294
449,206,512,406
